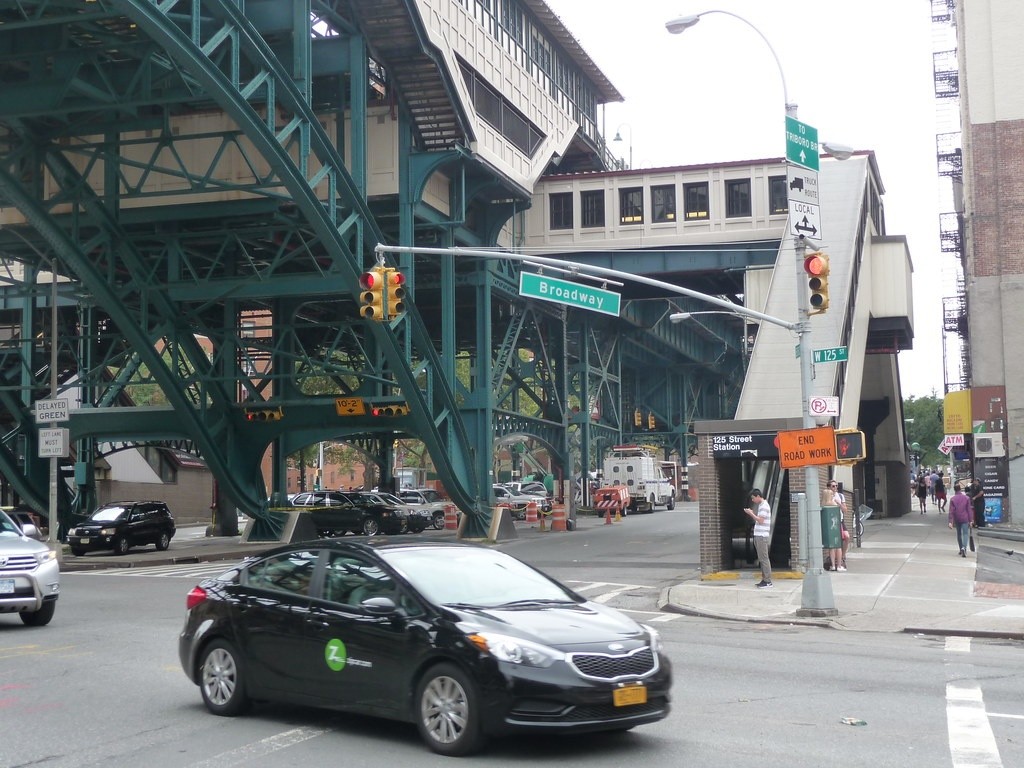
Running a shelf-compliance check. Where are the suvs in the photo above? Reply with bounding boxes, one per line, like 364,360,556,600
267,481,552,536
0,506,62,626
66,500,177,556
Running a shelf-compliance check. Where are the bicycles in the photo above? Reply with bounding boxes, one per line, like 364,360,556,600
852,511,865,538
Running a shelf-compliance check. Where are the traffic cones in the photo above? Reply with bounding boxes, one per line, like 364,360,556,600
614,507,622,522
604,508,613,525
537,514,549,532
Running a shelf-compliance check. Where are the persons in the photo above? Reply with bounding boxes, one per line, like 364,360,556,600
822,479,850,571
916,476,927,514
948,484,974,557
935,472,947,513
339,485,344,490
743,489,773,588
910,468,938,505
972,478,985,528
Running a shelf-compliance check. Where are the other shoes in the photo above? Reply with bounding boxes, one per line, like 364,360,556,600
837,566,847,571
959,548,966,557
829,566,835,570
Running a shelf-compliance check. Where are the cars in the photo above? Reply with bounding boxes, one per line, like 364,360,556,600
178,534,674,755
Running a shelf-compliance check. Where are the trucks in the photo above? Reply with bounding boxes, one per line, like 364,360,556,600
593,448,676,518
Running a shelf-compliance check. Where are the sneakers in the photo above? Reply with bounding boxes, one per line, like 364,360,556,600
755,579,773,588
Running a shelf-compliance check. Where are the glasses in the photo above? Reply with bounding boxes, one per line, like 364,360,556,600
830,484,837,487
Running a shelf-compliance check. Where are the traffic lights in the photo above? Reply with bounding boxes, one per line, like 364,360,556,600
635,412,641,426
371,405,409,418
648,415,656,430
386,267,406,316
804,253,829,310
246,411,282,422
360,268,383,319
835,431,867,461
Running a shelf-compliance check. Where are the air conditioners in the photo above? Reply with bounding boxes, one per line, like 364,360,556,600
973,432,1005,459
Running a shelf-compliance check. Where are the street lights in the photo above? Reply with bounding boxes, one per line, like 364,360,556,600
911,442,920,475
666,9,853,617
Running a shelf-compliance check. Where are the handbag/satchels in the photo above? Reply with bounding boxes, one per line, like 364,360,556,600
969,536,975,552
